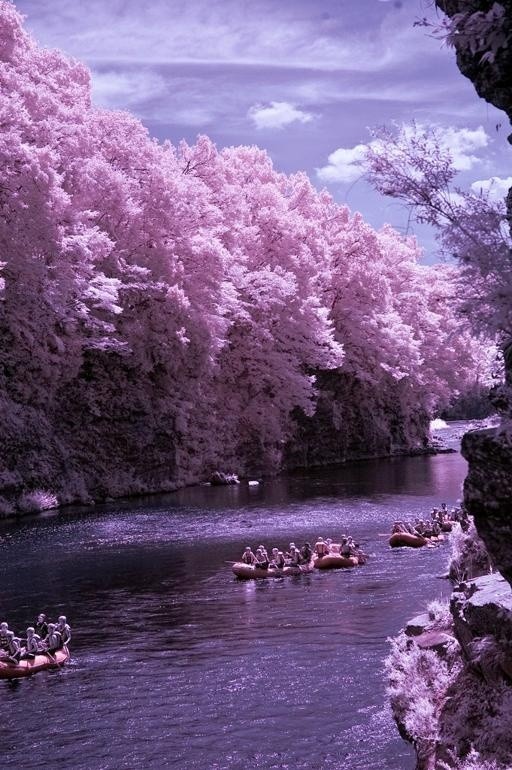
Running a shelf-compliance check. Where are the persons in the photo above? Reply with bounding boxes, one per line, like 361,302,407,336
421,434,428,448
391,502,472,538
0,613,71,664
241,534,356,569
211,470,238,481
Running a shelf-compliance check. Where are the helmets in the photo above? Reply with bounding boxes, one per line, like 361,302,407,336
6,632,14,639
342,534,352,541
47,624,55,632
245,543,264,553
272,548,278,554
395,518,438,525
318,536,332,542
305,542,310,548
431,503,464,517
27,627,34,634
289,543,295,549
1,622,8,629
39,614,46,621
58,616,66,623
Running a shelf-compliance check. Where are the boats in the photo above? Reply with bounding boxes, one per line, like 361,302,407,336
312,543,367,569
389,515,459,548
1,643,71,678
208,474,239,485
231,556,315,578
